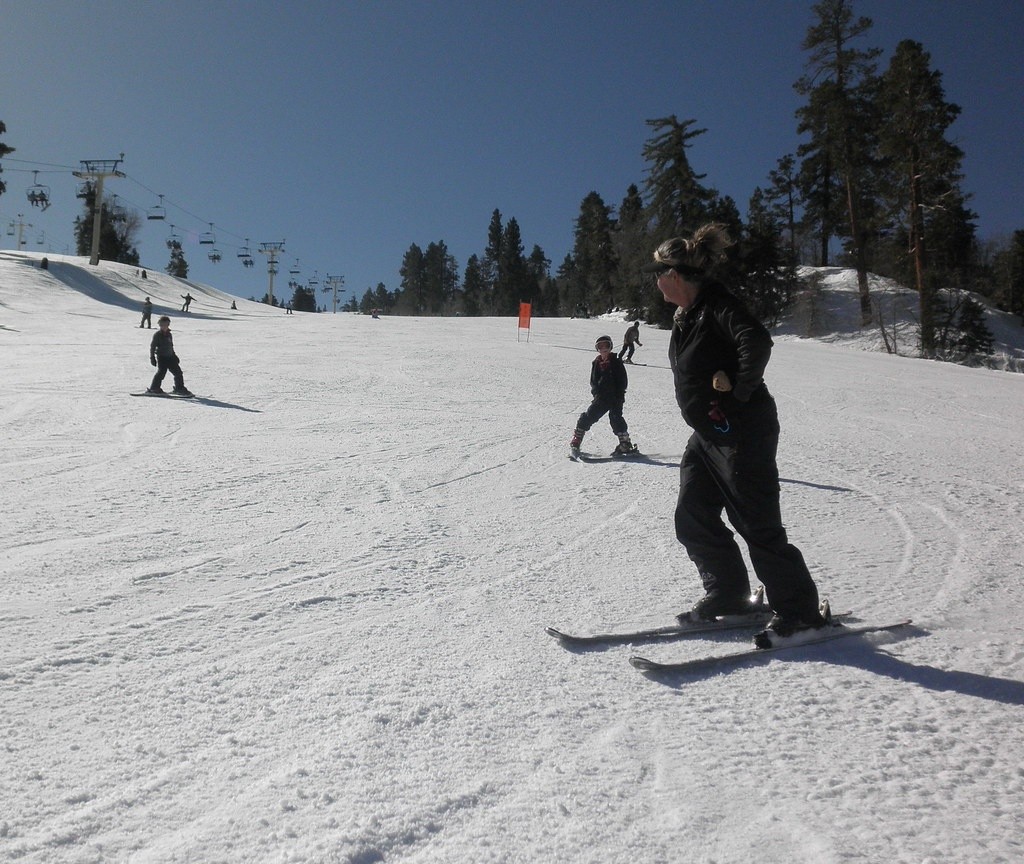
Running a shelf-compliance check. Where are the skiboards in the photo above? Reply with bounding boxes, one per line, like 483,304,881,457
565,444,662,463
542,602,915,685
622,361,648,367
129,387,214,400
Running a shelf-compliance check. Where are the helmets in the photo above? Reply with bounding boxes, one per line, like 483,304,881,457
158,317,170,324
594,335,613,351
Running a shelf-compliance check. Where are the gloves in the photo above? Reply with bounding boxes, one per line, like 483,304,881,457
150,356,157,366
176,356,179,363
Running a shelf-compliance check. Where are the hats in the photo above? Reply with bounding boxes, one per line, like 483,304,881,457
640,257,704,274
635,321,640,325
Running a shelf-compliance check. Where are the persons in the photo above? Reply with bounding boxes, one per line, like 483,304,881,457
140,297,152,329
30,190,48,207
136,269,139,276
569,336,631,450
150,316,187,391
286,300,293,315
231,300,237,309
168,240,176,246
570,300,591,319
372,308,377,317
640,222,821,647
80,180,91,193
288,280,297,289
181,293,197,312
212,254,220,264
619,322,643,363
244,259,253,268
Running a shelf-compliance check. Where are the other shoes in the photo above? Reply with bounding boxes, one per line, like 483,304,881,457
625,358,633,364
147,387,162,394
174,388,190,395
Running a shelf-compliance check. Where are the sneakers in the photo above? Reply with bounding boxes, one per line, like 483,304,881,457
677,594,750,625
615,437,632,454
571,429,584,448
755,611,824,647
